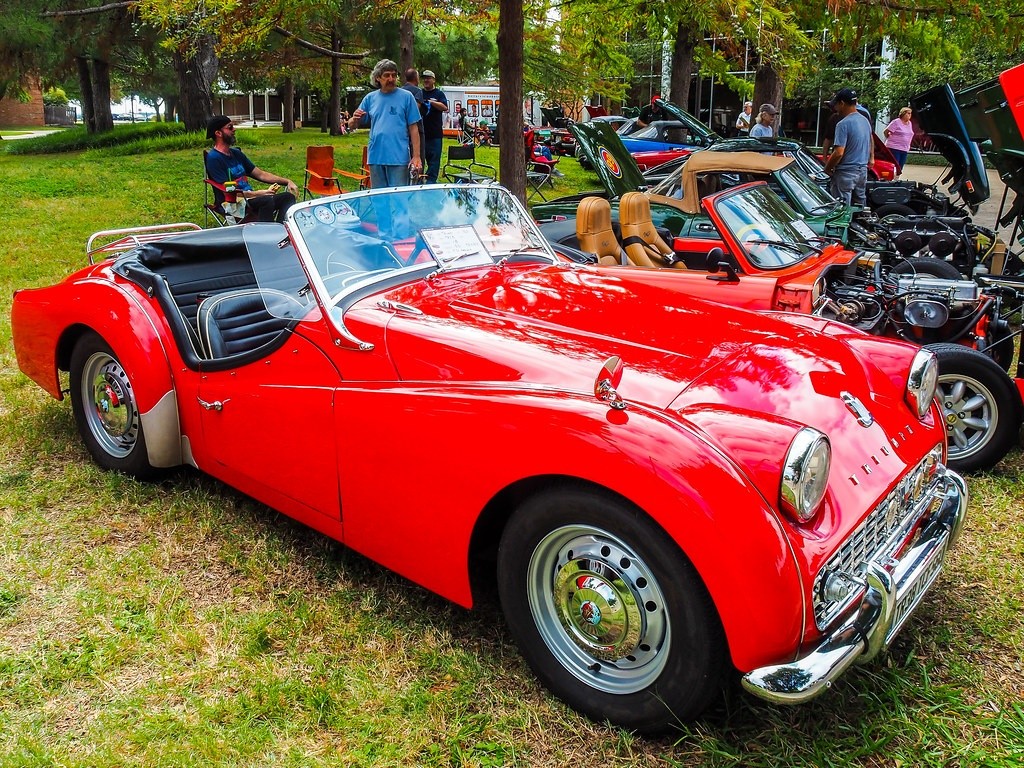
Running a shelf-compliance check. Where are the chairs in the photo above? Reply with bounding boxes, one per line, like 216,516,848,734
442,145,497,184
524,130,560,184
203,147,281,229
619,191,687,268
525,146,551,203
198,287,304,360
458,117,481,147
303,146,370,203
306,270,368,300
360,146,427,190
577,196,637,266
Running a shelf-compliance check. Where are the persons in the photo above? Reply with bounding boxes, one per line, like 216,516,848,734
884,107,914,181
469,105,478,116
822,86,872,210
483,106,493,117
454,104,460,117
340,58,493,243
637,95,667,129
736,100,753,136
206,115,299,225
750,104,779,156
524,123,564,177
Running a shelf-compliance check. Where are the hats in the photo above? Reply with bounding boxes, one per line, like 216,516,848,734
206,115,231,140
652,95,661,103
829,89,857,102
420,70,435,79
760,104,780,115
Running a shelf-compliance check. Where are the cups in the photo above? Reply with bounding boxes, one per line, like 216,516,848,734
224,181,237,192
409,164,419,185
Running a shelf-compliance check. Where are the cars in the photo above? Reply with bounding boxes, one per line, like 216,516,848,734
525,97,1024,303
397,179,1024,476
11,177,969,737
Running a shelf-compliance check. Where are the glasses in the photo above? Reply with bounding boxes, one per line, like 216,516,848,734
222,124,234,130
